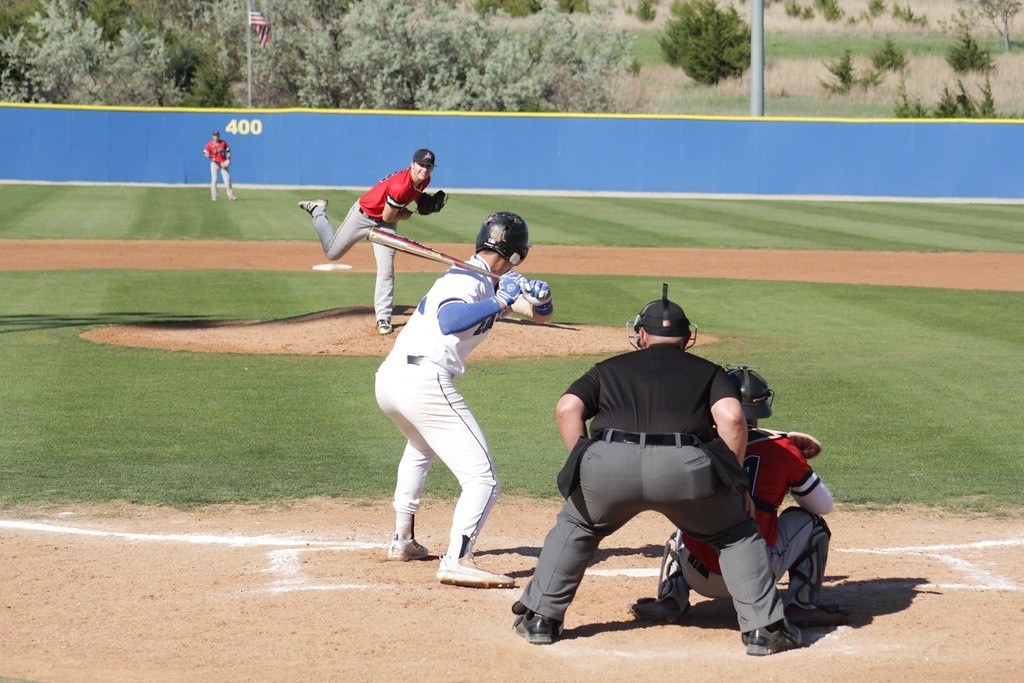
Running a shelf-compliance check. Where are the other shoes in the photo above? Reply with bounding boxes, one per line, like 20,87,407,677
212,196,218,203
229,195,237,201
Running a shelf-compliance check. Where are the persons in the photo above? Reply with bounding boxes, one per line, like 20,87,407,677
298,148,448,334
375,211,551,589
202,130,237,201
512,282,800,656
626,364,850,628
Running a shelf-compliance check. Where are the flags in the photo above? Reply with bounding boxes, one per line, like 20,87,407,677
249,4,268,49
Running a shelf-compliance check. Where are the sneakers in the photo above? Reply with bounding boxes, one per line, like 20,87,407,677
788,605,851,628
515,612,553,644
630,600,673,623
438,558,517,588
747,624,802,656
388,540,428,560
298,200,326,218
377,320,393,336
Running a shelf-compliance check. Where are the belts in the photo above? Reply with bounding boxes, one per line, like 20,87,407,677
360,209,381,224
409,357,422,365
688,555,713,579
601,430,696,447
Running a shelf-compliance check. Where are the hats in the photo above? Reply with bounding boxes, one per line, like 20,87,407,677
213,130,220,135
413,149,436,168
639,299,688,336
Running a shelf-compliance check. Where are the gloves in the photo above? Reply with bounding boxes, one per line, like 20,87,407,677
494,270,527,307
520,277,554,316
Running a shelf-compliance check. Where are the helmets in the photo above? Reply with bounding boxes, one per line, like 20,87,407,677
475,214,533,265
727,367,773,419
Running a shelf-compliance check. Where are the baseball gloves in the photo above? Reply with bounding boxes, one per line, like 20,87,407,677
220,159,230,168
415,189,449,215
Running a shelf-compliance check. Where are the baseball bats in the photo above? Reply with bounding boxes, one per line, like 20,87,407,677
366,227,551,299
